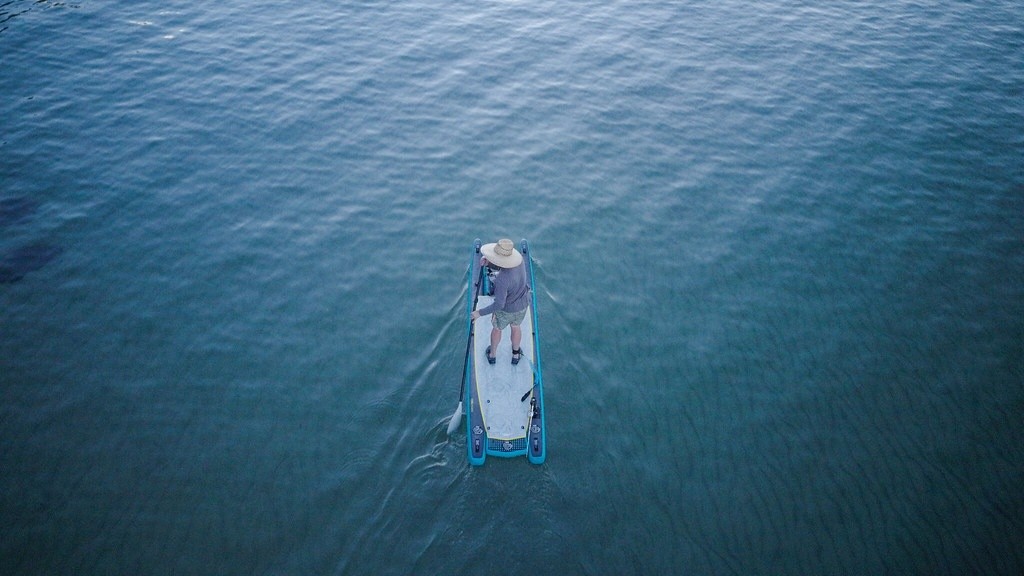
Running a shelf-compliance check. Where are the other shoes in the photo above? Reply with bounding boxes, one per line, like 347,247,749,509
485,345,497,364
511,346,524,364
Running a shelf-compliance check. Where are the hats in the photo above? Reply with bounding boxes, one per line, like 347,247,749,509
480,238,523,269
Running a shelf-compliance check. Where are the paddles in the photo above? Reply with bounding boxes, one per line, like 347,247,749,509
446,265,484,432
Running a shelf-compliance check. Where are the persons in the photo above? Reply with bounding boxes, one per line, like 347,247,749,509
469,238,528,365
